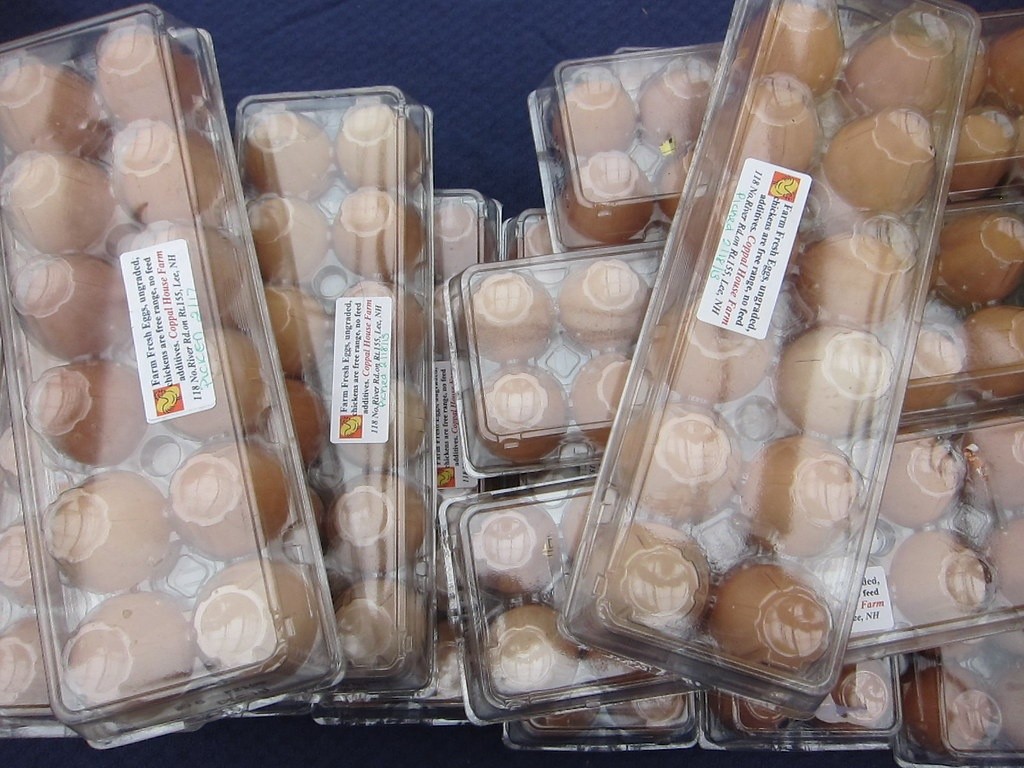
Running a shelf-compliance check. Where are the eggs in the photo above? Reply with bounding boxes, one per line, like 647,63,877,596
0,0,1024,768
